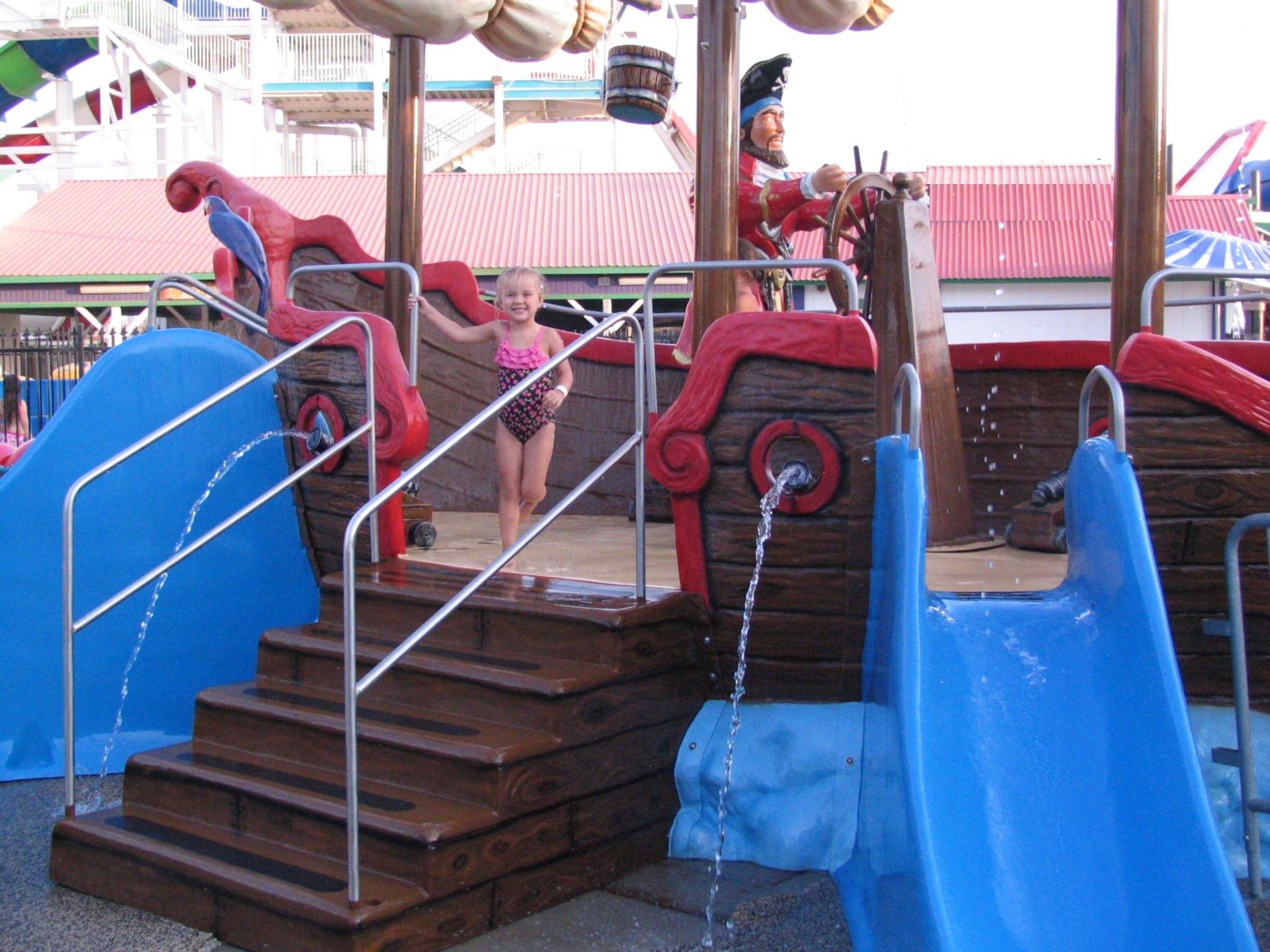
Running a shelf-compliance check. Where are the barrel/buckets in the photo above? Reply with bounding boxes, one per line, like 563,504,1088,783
604,44,674,125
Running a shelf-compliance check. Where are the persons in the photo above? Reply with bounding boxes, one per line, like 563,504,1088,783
407,265,573,571
0,374,32,445
670,52,931,368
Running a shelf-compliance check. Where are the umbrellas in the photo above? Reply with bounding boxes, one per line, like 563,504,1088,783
1165,229,1270,342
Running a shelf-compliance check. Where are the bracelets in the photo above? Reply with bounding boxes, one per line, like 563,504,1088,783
554,385,568,397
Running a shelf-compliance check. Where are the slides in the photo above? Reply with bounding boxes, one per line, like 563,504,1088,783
876,362,1255,950
